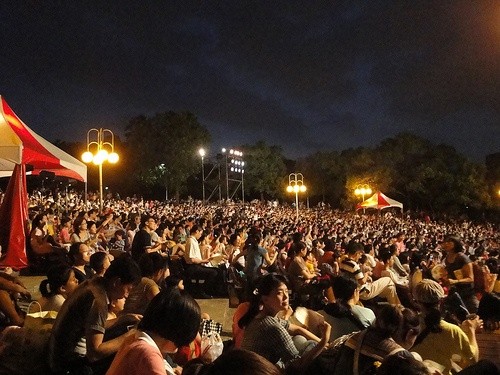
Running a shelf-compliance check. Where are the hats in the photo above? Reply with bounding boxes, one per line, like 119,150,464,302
416,278,444,302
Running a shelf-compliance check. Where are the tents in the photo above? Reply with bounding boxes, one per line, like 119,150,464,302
355,190,403,223
0,95,87,205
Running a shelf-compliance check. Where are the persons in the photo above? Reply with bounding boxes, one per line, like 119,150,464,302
0,189,500,375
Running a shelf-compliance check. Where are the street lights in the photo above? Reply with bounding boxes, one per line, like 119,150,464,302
199,147,206,205
354,184,372,214
287,173,306,219
82,127,119,212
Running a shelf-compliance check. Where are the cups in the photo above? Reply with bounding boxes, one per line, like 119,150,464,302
466,314,483,334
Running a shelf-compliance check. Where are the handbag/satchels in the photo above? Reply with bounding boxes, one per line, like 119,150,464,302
200,331,224,363
21,301,65,357
199,319,223,340
